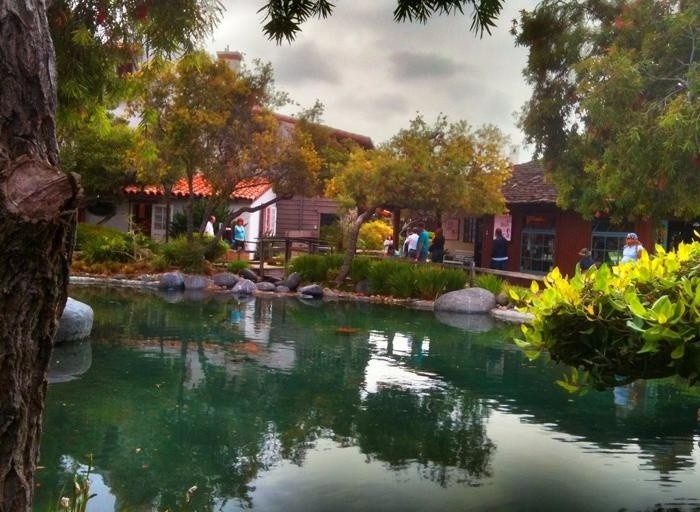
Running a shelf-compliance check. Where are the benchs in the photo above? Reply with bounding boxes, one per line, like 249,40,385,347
443,249,476,266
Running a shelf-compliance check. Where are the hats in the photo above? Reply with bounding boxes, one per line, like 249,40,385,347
579,248,592,256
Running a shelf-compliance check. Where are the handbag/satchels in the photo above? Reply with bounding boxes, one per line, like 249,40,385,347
388,245,394,254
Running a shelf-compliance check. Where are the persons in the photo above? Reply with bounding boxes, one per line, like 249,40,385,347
490,229,509,271
203,215,217,238
230,218,246,251
578,247,593,274
383,222,445,264
612,376,635,422
230,302,240,332
621,233,643,265
